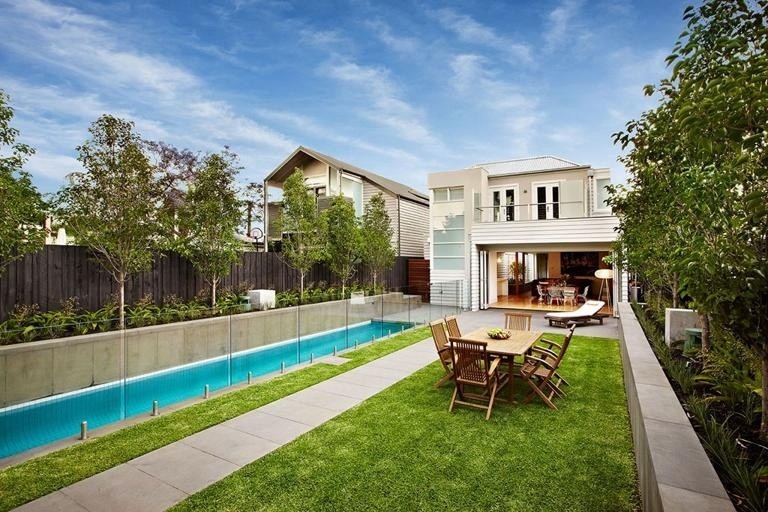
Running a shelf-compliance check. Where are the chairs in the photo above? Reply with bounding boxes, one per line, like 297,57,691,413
537,280,590,307
544,299,611,329
427,311,577,421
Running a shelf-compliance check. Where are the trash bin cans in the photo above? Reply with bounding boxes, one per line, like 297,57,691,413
684,328,702,350
237,296,252,312
